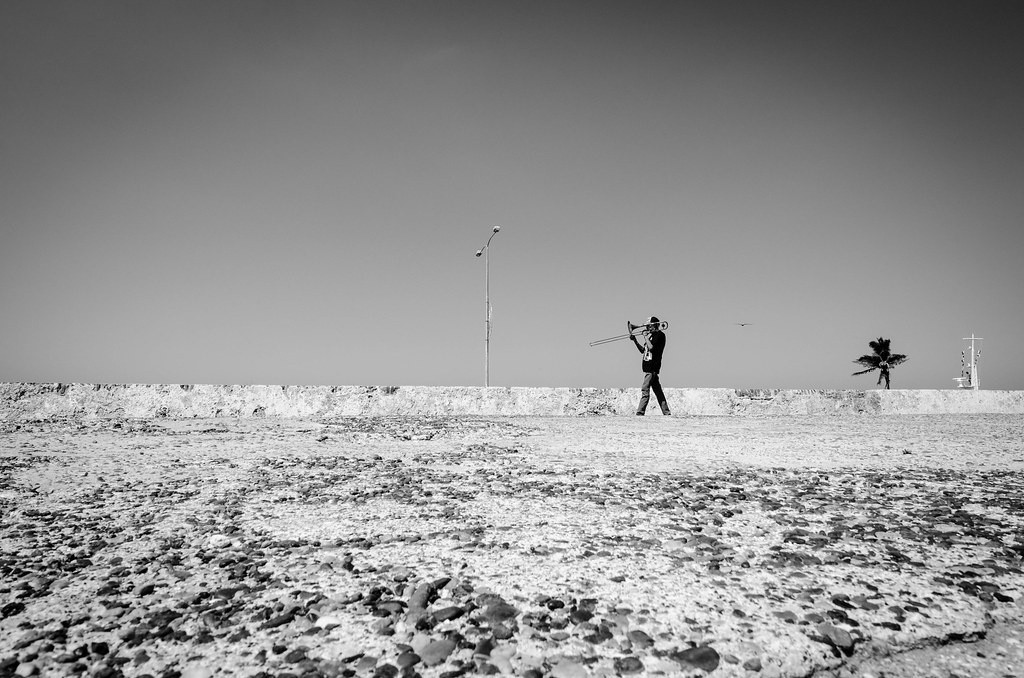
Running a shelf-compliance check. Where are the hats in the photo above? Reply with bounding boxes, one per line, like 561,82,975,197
644,316,660,324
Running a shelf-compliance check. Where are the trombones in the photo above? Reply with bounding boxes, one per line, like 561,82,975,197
589,320,669,347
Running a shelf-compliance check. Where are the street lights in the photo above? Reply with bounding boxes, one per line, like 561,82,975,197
475,225,502,389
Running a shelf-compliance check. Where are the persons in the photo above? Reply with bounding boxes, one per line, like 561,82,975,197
630,317,672,416
